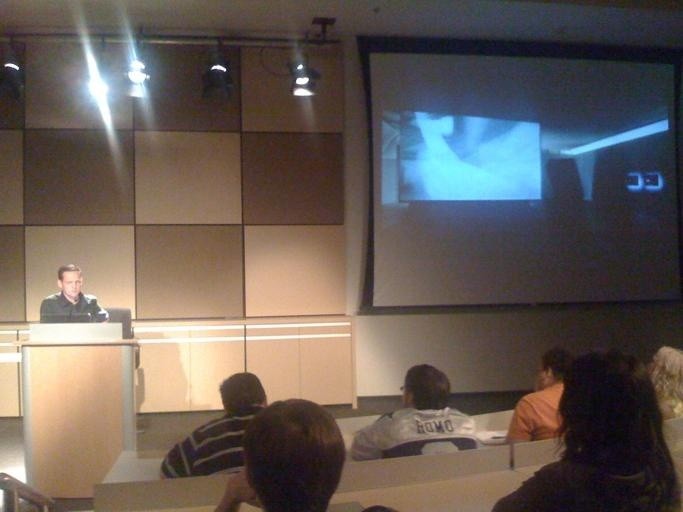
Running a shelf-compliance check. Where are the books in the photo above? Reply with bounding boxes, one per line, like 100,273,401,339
477,430,508,444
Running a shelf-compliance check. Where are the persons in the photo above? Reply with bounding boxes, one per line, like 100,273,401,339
497,345,572,443
641,343,683,425
38,263,110,322
349,363,477,460
158,371,269,480
488,346,682,511
201,397,347,511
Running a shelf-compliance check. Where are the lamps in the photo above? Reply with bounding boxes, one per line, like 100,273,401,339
81,56,109,99
197,58,237,100
4,55,26,100
121,47,152,99
286,52,317,99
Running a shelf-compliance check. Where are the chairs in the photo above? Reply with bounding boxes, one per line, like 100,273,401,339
93,410,683,512
